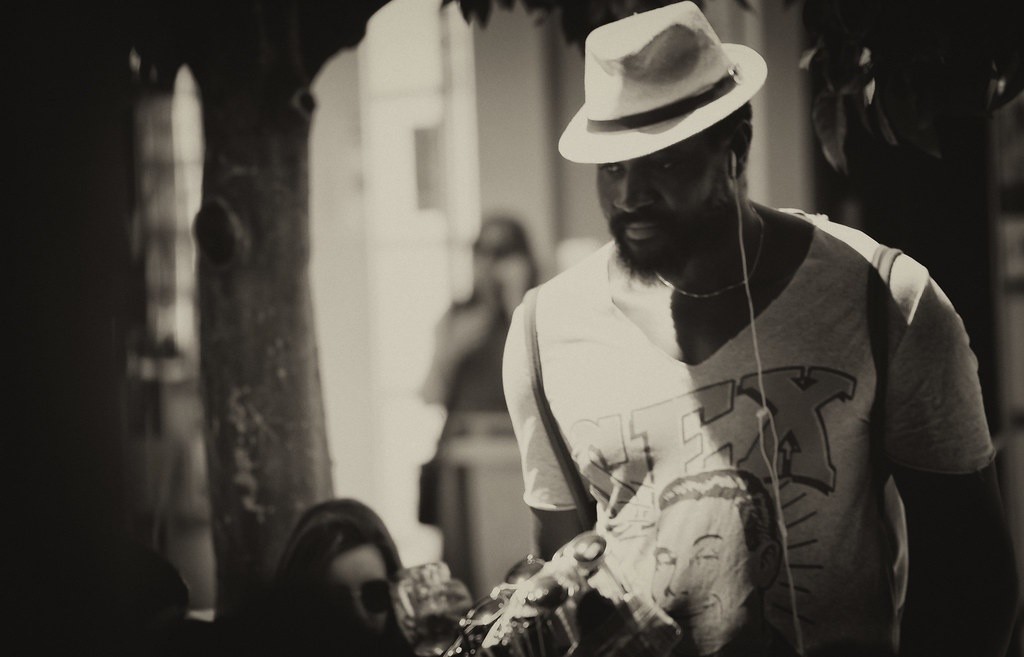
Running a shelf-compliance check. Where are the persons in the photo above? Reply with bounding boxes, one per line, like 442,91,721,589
502,2,1016,657
423,211,541,602
254,500,402,657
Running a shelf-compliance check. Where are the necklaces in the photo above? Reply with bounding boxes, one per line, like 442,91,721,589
655,205,766,298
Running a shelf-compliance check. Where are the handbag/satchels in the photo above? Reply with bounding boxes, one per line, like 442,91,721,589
420,460,442,523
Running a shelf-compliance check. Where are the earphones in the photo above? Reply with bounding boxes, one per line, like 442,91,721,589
728,147,740,181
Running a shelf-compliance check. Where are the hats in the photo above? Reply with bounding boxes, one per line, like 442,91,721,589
558,0,767,163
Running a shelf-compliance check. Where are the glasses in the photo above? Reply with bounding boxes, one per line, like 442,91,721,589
332,580,390,613
